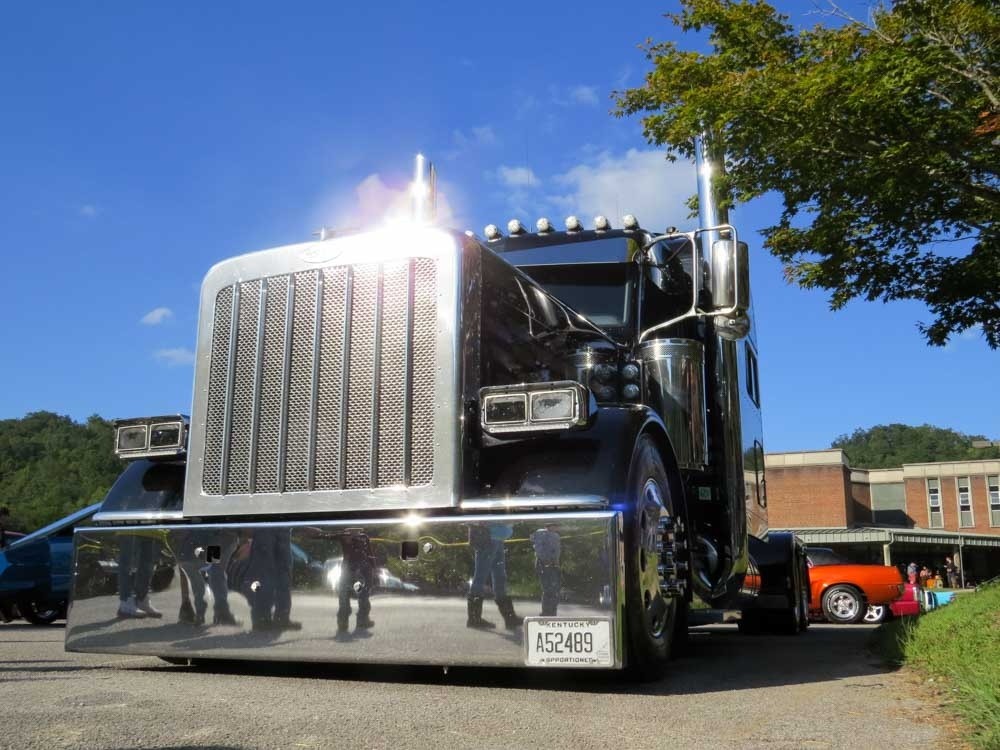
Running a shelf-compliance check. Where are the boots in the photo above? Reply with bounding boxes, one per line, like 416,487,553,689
466,596,497,628
135,598,163,618
117,597,147,618
494,596,525,628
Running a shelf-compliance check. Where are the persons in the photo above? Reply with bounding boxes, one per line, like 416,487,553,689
907,561,916,584
944,557,958,588
336,539,375,631
165,528,245,626
532,523,561,616
920,566,932,578
114,528,168,619
467,524,524,628
252,528,303,630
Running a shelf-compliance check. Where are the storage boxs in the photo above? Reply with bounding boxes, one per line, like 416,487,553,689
934,591,955,606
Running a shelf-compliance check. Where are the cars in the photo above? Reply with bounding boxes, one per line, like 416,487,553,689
747,547,904,621
0,504,104,624
863,583,924,623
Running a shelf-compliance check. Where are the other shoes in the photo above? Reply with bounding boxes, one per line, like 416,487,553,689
357,621,375,629
272,619,303,630
336,619,349,631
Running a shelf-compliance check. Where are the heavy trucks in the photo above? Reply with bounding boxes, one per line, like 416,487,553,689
67,122,812,679
